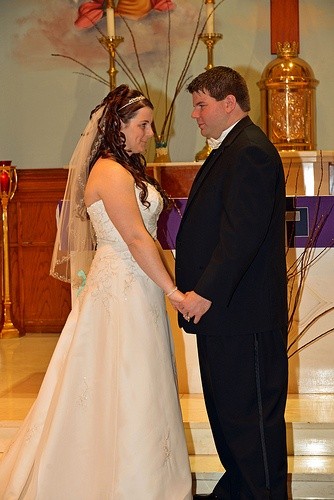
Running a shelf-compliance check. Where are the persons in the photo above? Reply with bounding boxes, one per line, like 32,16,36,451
173,67,290,500
80,84,195,500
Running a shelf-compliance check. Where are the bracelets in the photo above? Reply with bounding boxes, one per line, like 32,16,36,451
166,286,178,297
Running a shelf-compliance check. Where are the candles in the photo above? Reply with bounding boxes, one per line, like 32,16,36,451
106,0,115,36
205,0,215,33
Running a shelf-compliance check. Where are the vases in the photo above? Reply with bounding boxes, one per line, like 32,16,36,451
153,135,171,162
